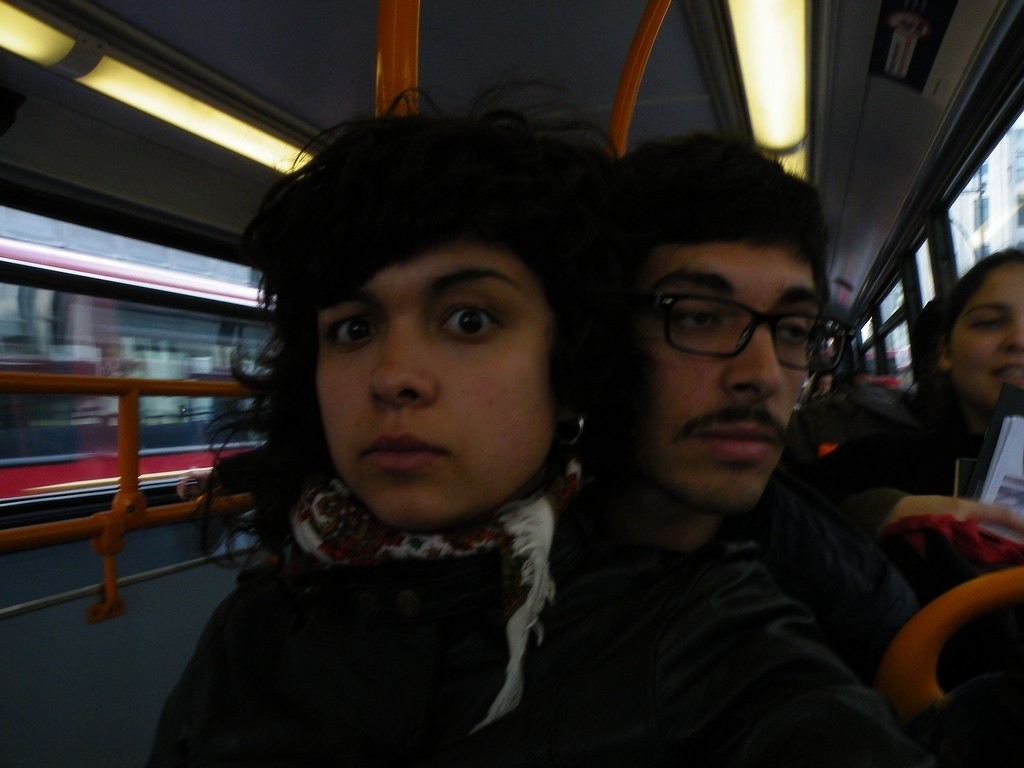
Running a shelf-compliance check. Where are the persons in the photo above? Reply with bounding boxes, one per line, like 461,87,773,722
145,86,1024,768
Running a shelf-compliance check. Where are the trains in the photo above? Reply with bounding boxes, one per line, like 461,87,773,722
0,234,291,526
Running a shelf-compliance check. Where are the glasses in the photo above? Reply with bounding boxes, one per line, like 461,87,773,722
650,291,858,372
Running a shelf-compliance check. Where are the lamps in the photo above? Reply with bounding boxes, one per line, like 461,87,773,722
0,0,831,182
